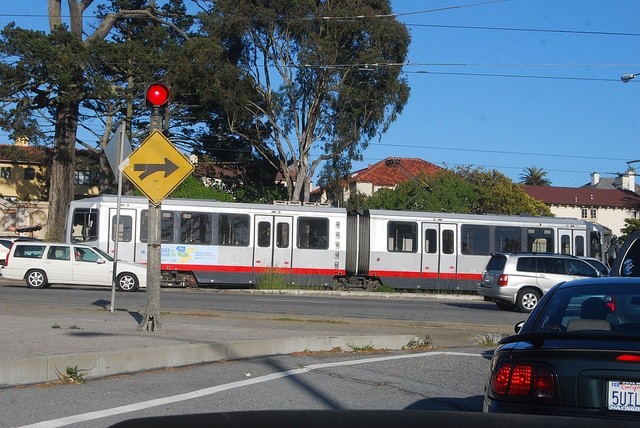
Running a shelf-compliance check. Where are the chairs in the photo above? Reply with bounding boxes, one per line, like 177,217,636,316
567,320,611,331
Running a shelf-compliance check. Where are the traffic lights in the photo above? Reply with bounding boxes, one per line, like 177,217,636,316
141,80,173,124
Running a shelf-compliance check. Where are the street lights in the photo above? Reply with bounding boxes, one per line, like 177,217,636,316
621,70,640,83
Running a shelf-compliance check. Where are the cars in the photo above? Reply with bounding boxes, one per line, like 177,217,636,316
578,254,610,276
0,235,41,266
482,279,638,414
610,228,639,277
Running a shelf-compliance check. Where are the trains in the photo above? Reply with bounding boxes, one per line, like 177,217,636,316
65,192,615,291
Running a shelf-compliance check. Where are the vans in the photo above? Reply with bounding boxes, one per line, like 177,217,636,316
1,239,162,292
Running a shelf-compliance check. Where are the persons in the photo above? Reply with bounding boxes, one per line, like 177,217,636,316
581,297,608,320
75,251,83,260
79,250,86,259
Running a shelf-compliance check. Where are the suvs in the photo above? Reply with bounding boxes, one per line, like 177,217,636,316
477,250,611,312
